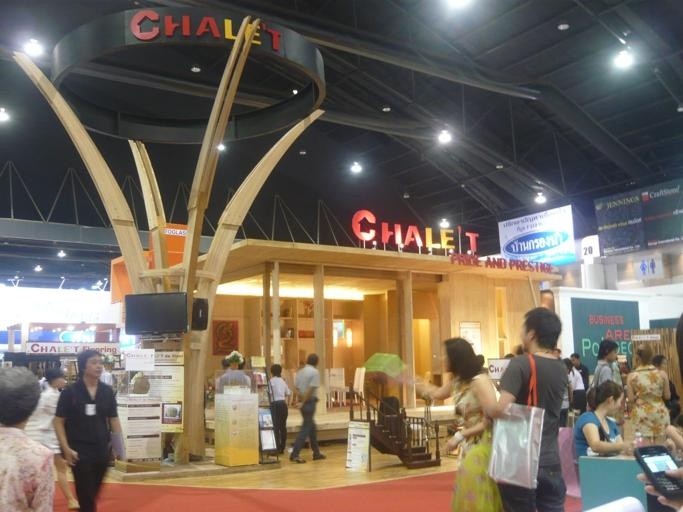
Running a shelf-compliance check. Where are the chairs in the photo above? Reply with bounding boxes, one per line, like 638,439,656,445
557,427,581,499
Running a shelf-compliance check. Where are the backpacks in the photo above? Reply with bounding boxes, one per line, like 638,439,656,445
586,364,613,409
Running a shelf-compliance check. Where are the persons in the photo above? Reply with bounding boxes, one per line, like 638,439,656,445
637,313,682,512
53,349,126,512
213,357,234,384
474,305,570,512
100,356,115,387
266,363,293,457
288,352,328,464
216,356,251,392
504,337,683,487
0,364,56,512
23,365,81,510
394,336,505,512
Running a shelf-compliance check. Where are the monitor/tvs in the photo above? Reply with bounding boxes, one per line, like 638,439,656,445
125,292,187,339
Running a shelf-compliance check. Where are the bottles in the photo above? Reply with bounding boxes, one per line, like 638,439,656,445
632,433,644,449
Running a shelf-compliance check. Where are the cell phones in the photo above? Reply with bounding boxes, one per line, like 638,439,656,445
634,444,683,500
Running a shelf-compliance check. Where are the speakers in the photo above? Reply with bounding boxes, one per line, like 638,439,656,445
192,297,208,331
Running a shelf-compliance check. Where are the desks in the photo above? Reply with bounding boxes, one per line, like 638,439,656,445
579,456,649,512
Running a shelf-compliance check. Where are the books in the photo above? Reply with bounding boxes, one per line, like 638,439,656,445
253,370,277,453
109,431,126,462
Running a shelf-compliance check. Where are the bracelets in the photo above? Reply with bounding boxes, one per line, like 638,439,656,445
455,431,462,441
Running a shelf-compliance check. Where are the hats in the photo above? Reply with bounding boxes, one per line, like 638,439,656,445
0,366,41,424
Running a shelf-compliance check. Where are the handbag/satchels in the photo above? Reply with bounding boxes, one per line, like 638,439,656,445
488,353,545,489
